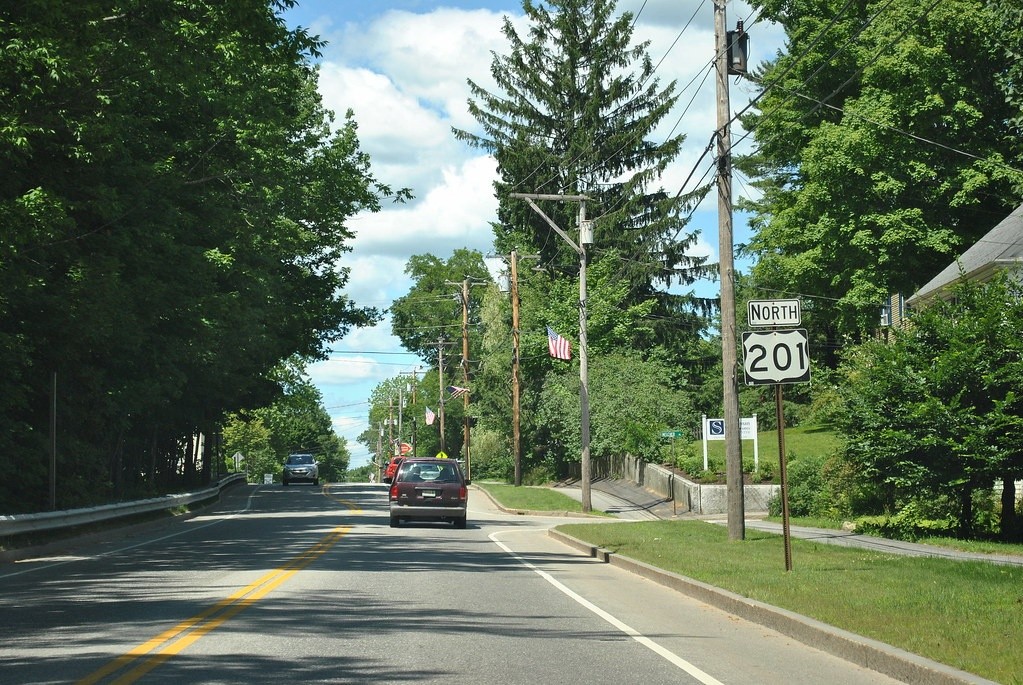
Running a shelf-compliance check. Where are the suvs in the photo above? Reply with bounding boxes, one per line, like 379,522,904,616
281,453,320,486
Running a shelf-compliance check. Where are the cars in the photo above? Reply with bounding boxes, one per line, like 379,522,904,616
384,456,405,484
389,457,471,527
411,464,441,481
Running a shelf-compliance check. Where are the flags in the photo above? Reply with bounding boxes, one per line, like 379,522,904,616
548,327,571,359
446,386,469,398
426,407,435,425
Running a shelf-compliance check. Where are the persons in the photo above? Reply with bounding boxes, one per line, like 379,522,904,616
369,471,374,483
